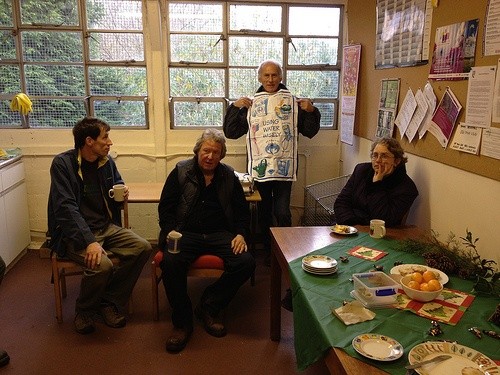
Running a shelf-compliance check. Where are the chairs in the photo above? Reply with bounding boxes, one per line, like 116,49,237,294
42,226,130,326
148,246,233,325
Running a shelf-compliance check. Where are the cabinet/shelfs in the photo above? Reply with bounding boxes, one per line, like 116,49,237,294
0,150,32,278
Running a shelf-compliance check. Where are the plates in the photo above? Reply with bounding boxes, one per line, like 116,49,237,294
408,341,500,375
352,333,404,362
302,255,338,275
390,264,450,285
329,225,358,235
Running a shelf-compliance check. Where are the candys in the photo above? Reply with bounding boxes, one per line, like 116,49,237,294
340,255,401,281
428,320,500,345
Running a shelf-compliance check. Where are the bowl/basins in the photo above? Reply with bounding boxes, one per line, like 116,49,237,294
400,277,443,302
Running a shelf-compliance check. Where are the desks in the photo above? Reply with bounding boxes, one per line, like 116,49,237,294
269,225,500,375
118,179,262,287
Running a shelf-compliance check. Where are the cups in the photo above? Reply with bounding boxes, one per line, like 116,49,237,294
109,184,125,201
369,219,386,238
167,230,182,254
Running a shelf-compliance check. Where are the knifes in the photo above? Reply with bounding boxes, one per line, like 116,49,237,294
405,355,452,369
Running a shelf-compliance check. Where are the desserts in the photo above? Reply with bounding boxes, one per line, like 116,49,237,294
398,265,439,279
462,366,485,375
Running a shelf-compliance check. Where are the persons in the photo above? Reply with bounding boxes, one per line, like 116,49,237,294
158,128,255,351
334,138,417,227
47,118,153,334
223,61,321,226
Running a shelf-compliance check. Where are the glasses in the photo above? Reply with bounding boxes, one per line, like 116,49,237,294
369,153,394,161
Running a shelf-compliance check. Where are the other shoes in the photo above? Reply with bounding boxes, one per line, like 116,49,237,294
95,301,127,328
194,304,227,337
74,313,95,335
166,323,193,354
0,350,10,368
264,255,271,266
282,288,293,313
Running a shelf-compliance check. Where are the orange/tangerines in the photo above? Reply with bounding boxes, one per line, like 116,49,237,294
402,270,441,291
